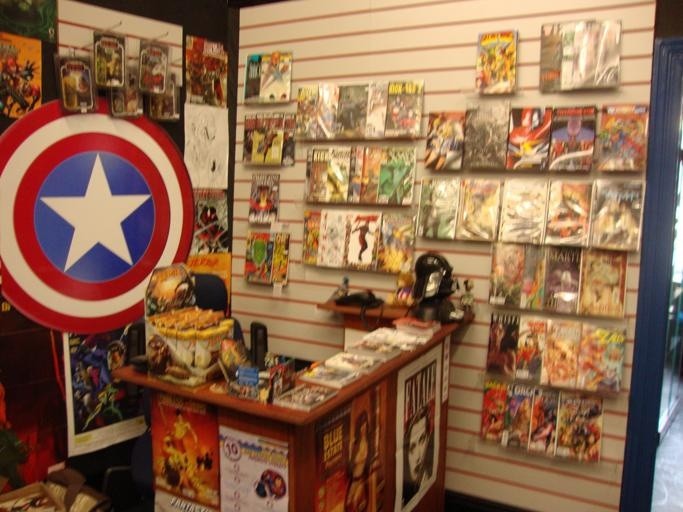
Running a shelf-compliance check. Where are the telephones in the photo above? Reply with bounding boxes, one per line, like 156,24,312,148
336,290,380,309
